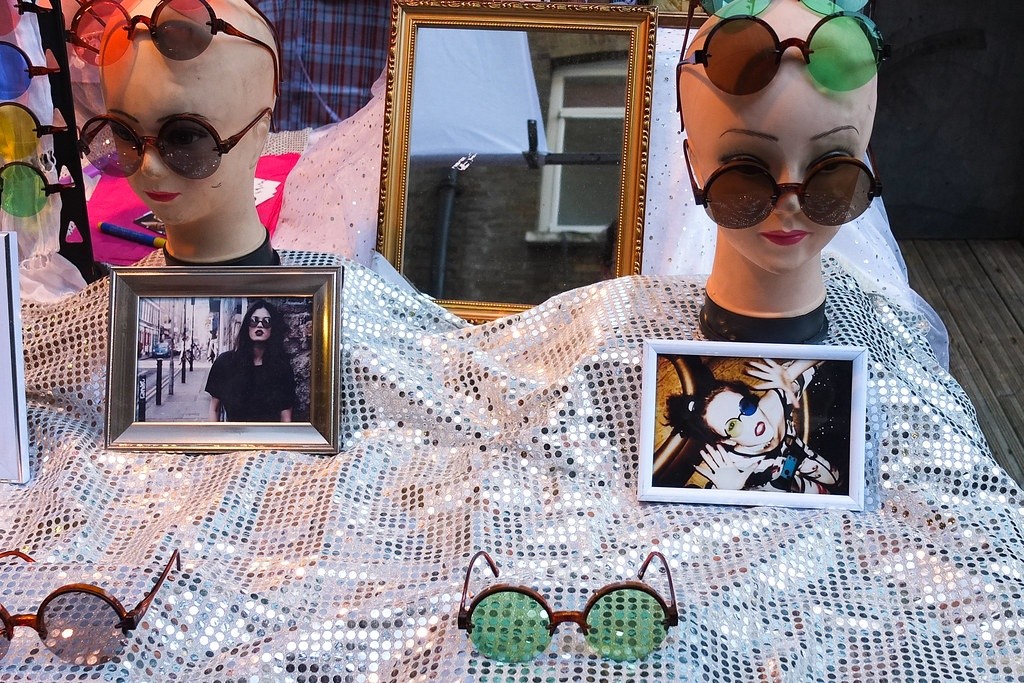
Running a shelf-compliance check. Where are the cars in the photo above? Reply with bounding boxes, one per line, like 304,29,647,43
152,342,170,358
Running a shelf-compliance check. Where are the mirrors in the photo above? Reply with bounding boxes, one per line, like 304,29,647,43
375,0,658,327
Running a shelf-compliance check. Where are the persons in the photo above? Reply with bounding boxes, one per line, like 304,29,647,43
659,357,846,496
205,301,296,421
99,0,282,272
677,0,878,343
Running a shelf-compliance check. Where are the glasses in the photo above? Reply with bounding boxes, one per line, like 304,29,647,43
79,107,278,180
675,11,888,133
456,550,681,663
0,0,83,219
64,0,282,97
682,0,873,58
683,137,884,229
248,316,274,329
721,394,758,440
0,549,189,667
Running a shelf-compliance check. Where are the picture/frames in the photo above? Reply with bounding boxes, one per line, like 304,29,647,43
637,339,869,510
104,265,345,456
